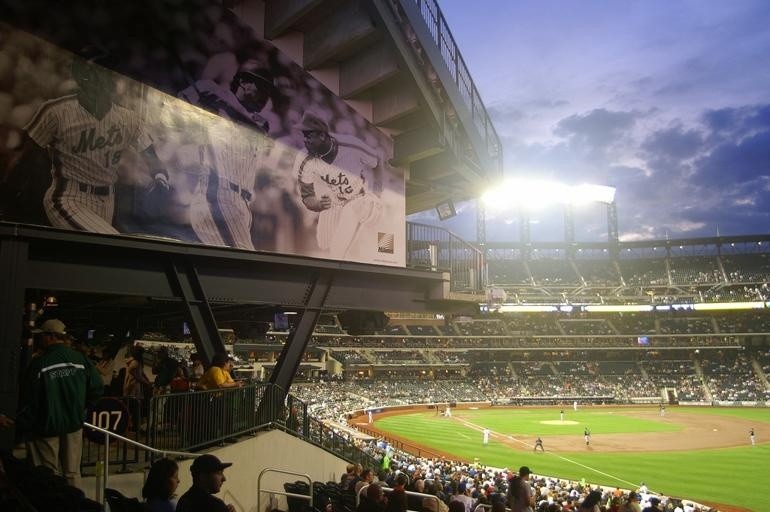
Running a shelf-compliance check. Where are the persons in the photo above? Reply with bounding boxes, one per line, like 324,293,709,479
1,23,385,258
0,271,770,511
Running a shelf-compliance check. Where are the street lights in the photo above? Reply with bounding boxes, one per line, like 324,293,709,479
473,176,620,263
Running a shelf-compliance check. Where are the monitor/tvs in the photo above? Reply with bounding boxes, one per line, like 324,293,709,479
274,313,289,330
183,322,190,335
638,336,649,345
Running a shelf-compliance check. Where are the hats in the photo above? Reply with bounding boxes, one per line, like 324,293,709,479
520,466,532,474
191,455,233,472
32,320,64,335
